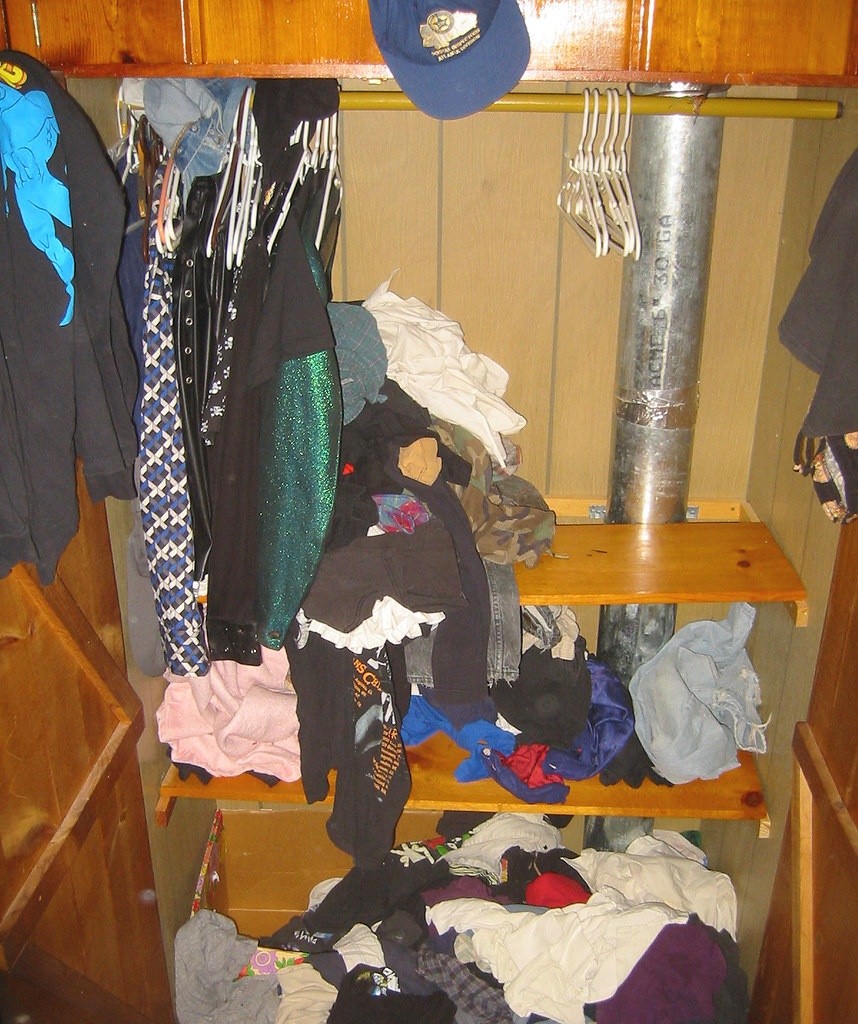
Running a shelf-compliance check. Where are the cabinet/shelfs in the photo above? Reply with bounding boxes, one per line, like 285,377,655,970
154,497,812,943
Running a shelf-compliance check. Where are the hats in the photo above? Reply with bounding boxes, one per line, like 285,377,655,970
491,634,592,745
368,0,531,121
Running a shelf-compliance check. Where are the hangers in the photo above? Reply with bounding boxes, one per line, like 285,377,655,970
96,81,644,274
